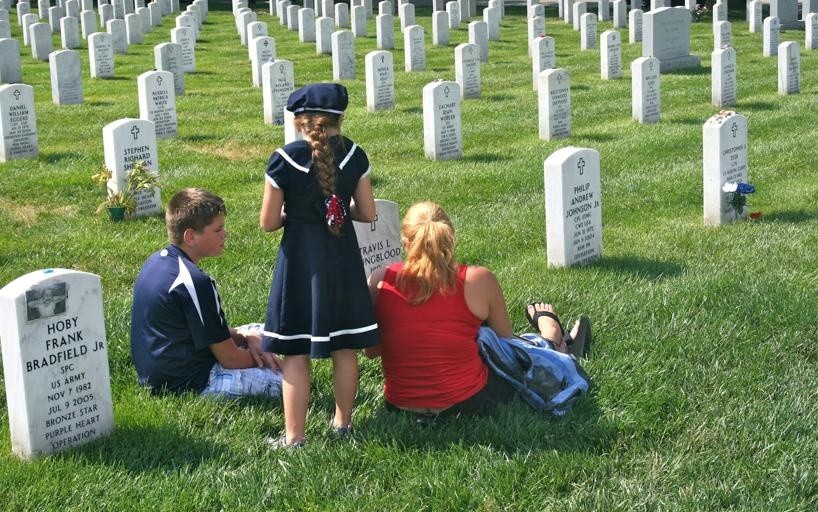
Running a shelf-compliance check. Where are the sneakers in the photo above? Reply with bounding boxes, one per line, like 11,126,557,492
331,422,352,435
265,436,305,452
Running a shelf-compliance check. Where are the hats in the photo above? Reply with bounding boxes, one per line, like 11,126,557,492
286,84,348,115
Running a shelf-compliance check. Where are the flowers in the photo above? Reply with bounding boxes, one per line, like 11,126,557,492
722,181,755,221
90,159,162,218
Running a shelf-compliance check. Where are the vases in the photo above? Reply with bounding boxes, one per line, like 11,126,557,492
106,207,125,224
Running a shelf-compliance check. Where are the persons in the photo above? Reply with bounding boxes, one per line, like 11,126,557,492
130,186,284,399
260,81,382,456
26,289,66,319
361,200,594,428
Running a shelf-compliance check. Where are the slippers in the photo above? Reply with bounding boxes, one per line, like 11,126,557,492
526,302,565,340
561,314,588,358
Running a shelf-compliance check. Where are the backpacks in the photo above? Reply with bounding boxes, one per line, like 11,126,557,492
480,325,590,419
